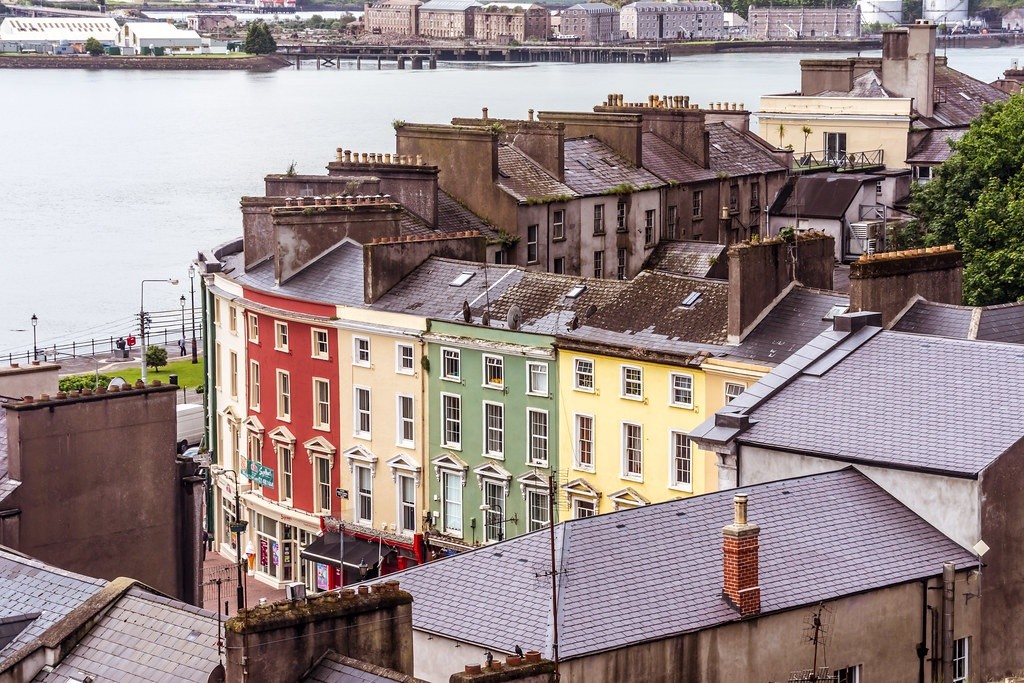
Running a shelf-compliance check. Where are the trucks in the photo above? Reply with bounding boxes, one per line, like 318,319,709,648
177,403,204,455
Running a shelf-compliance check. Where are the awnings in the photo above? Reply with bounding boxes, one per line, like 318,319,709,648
300,530,392,576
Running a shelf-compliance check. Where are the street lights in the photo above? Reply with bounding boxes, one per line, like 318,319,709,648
180,294,187,340
43,350,99,390
31,313,39,361
212,468,249,613
188,264,198,364
479,504,508,542
339,523,345,588
137,279,179,387
376,522,387,578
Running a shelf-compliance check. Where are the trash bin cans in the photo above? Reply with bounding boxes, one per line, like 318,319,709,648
169,374,178,384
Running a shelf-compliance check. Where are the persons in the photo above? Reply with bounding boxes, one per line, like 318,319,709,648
180,335,186,356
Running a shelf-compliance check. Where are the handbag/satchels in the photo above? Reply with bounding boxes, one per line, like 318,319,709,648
178,341,180,345
